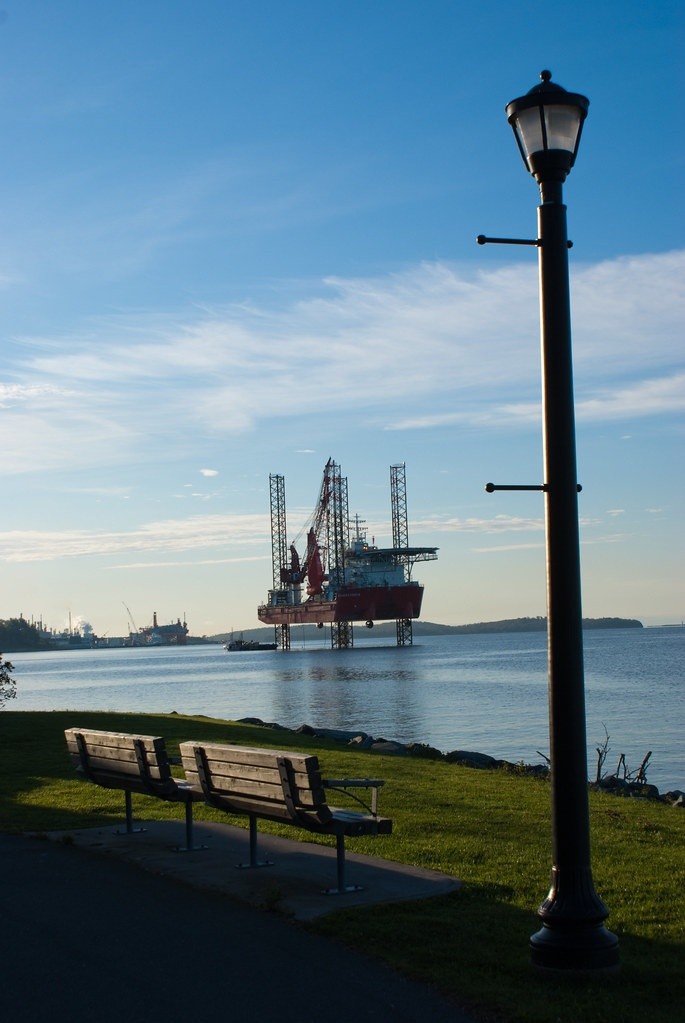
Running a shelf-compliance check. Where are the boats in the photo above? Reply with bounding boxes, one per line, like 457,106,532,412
224,638,279,651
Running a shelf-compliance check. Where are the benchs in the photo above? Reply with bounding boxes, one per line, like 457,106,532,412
63,726,208,852
178,739,392,894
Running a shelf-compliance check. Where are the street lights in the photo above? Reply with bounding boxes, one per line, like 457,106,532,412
475,69,624,974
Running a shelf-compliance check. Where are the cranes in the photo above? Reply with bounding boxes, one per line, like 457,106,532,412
276,456,338,604
